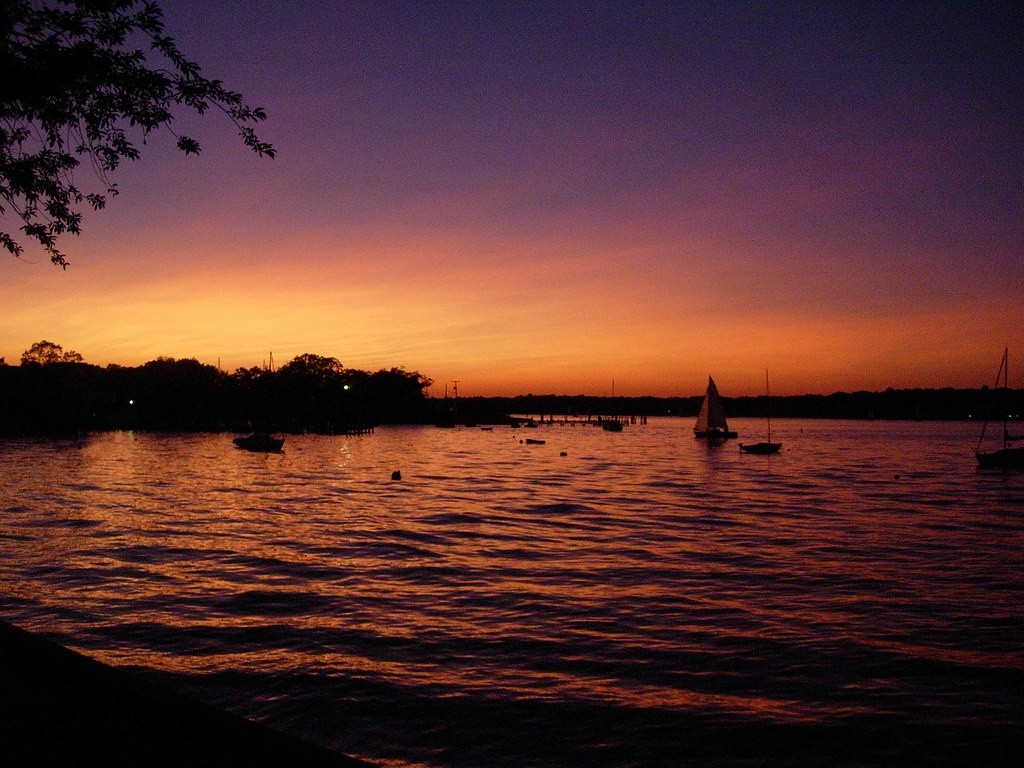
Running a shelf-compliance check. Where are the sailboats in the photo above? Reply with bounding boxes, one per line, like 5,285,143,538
974,347,1024,472
738,366,784,454
693,374,738,438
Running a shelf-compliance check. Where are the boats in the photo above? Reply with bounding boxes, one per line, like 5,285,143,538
231,431,285,453
600,418,624,434
